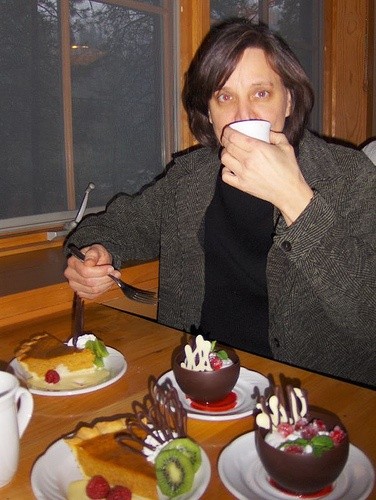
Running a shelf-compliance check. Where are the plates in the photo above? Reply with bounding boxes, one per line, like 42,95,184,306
7,345,128,396
217,430,374,499
153,366,274,420
31,431,212,500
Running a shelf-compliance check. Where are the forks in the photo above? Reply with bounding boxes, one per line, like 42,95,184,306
68,244,160,306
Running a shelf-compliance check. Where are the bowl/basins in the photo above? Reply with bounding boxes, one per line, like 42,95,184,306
175,345,240,402
255,409,350,493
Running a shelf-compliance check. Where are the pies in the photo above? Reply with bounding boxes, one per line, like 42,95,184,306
17,334,96,383
63,415,159,500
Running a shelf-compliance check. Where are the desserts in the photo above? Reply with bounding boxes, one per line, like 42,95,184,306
179,333,232,372
255,382,346,455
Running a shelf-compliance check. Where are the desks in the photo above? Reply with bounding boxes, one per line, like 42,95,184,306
0,302,376,500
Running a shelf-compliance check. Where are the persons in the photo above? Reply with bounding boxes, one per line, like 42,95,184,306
61,15,376,391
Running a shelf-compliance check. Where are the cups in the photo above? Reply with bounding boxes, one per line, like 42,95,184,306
228,120,271,145
0,371,34,486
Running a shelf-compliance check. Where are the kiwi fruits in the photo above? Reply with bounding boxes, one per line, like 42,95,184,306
86,339,109,367
155,449,194,497
163,438,202,474
